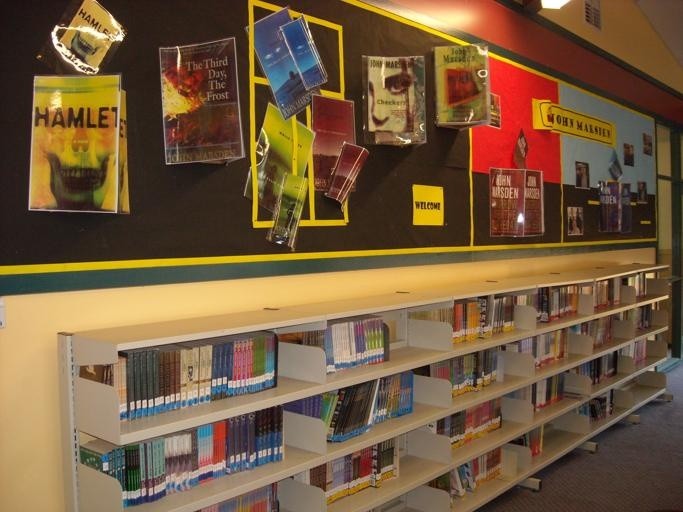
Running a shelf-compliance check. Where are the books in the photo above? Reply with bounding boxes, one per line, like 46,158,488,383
623,303,652,330
323,140,370,204
505,423,545,457
406,295,517,346
158,36,244,168
282,372,413,444
572,314,613,351
264,170,310,251
572,386,617,421
501,371,569,413
582,350,618,385
410,345,500,398
278,15,329,92
241,4,321,121
623,271,648,297
306,93,360,193
528,282,579,324
239,100,317,219
77,331,279,420
433,46,491,132
75,404,283,505
582,277,621,312
621,339,651,366
426,449,501,508
418,398,502,448
28,74,129,216
57,0,129,75
356,53,428,149
192,481,279,511
277,313,390,375
288,437,399,507
597,179,632,236
500,329,569,367
486,168,546,241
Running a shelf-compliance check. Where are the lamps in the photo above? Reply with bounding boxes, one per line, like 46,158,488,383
521,0,571,16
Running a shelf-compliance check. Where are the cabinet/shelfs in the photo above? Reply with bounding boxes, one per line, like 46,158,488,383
57,263,669,512
